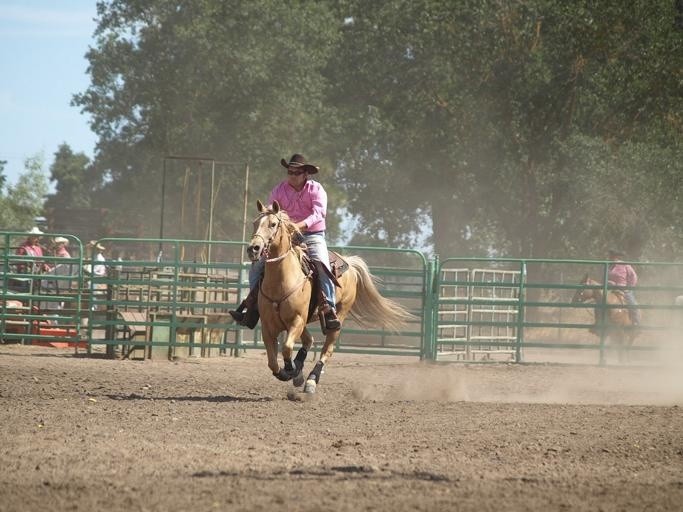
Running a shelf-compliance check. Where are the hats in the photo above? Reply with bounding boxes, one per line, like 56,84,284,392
85,240,105,251
280,154,317,174
606,241,627,258
24,227,43,241
51,236,68,246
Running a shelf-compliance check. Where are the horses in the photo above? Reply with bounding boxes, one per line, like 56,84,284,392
571,272,647,364
246,199,422,394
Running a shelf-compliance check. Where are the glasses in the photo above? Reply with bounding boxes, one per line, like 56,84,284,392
287,170,306,176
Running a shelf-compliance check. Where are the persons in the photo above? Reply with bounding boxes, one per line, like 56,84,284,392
12,226,50,316
42,236,74,324
80,240,107,312
228,151,341,331
591,247,641,331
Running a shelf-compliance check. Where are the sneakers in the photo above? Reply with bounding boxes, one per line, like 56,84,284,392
229,311,255,329
325,312,339,329
